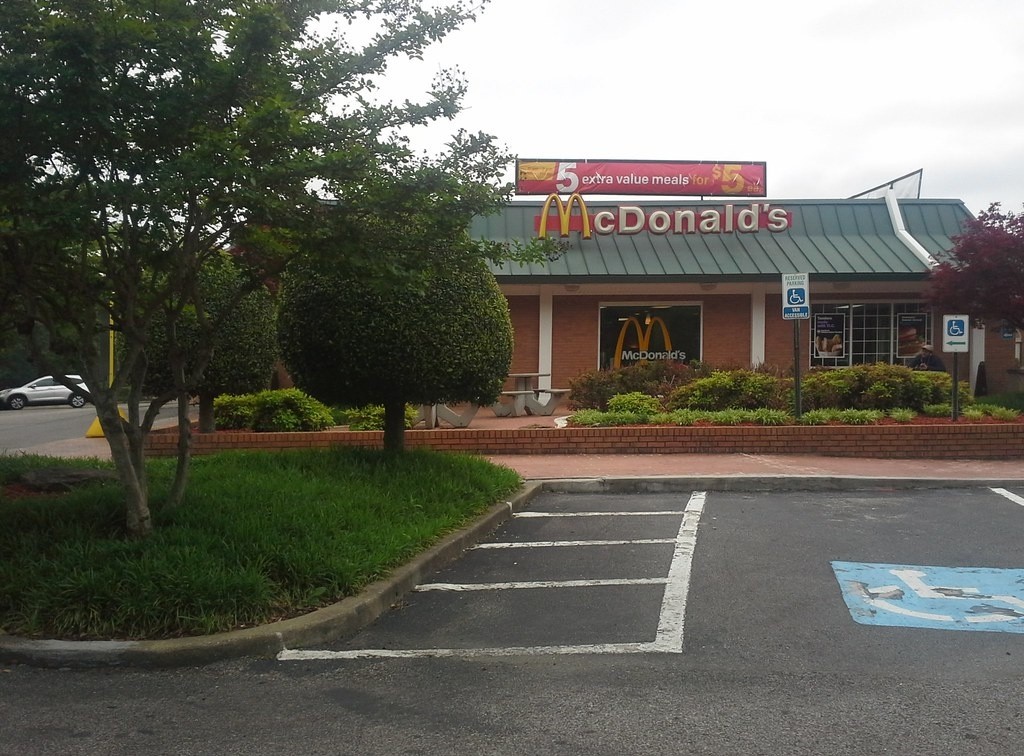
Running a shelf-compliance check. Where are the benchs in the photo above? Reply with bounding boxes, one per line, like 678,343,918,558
486,388,571,416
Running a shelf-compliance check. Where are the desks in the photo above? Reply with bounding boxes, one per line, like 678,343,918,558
504,372,551,416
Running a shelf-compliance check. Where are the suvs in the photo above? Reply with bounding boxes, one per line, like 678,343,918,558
0,375,91,410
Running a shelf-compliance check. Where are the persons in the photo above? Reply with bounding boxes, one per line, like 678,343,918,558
907,344,946,372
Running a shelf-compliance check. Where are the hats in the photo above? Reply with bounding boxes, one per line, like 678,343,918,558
921,345,934,352
